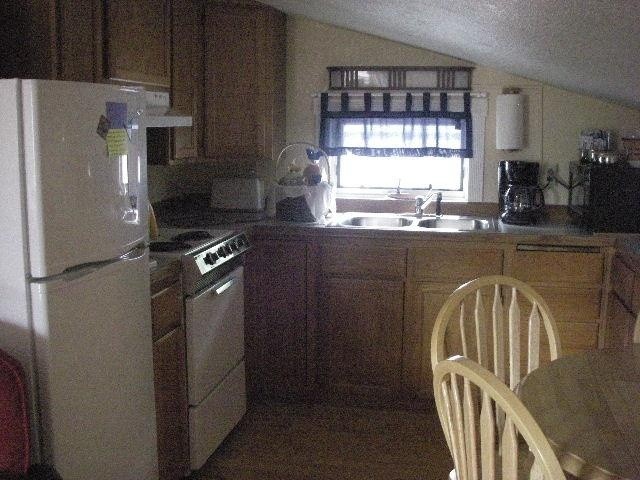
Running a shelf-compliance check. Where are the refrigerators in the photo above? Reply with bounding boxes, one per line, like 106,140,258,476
0,76,164,478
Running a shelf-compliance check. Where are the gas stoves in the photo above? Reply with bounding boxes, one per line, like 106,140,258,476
149,227,249,275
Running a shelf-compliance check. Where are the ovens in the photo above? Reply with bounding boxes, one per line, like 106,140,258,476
185,267,250,472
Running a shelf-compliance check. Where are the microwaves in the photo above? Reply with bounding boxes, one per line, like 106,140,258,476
564,160,639,231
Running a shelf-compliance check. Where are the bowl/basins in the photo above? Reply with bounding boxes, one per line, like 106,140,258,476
580,149,620,165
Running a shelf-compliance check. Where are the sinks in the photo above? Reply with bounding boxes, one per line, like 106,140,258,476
326,210,416,229
418,217,494,232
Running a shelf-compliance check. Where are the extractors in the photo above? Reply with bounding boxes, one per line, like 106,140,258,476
145,87,195,133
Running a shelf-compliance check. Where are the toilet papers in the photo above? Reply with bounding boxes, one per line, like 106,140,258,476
496,92,526,151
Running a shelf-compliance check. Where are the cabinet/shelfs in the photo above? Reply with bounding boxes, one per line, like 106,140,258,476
317,236,405,406
603,250,640,346
145,1,202,165
151,277,190,480
198,1,289,163
504,237,614,374
406,237,503,403
244,232,317,401
3,3,102,82
104,0,171,87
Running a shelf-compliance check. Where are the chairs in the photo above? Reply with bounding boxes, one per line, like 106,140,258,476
429,354,564,480
430,278,563,479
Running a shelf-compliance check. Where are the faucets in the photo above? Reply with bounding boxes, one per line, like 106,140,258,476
419,191,444,215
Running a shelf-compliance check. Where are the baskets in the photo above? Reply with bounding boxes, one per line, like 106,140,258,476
274,142,330,222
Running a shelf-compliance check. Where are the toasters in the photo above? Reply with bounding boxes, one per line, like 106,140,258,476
212,177,267,213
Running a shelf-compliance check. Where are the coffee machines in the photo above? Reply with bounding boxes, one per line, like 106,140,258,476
498,159,544,224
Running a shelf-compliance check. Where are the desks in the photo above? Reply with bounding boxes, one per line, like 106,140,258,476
514,345,639,480
